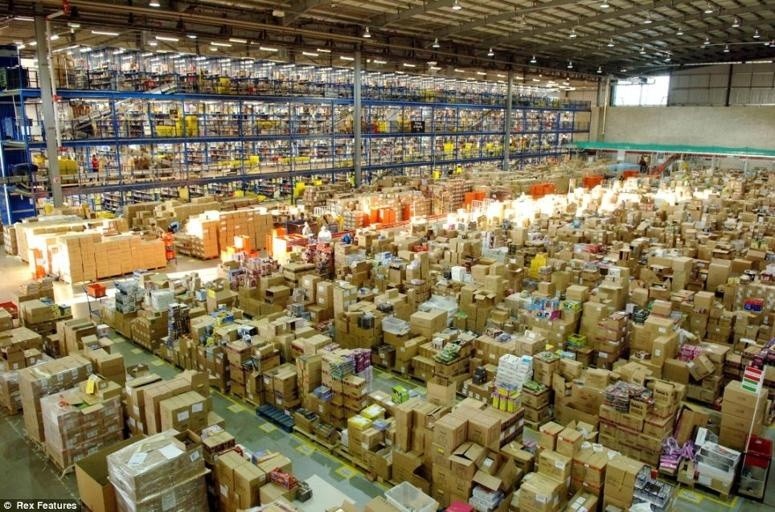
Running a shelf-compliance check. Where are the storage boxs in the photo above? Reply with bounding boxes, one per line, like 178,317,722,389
0,153,775,512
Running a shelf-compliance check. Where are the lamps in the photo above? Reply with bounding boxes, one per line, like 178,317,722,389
10,0,775,94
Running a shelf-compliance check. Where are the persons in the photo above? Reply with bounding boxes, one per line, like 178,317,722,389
300,221,313,237
299,212,309,222
639,157,647,174
88,155,99,183
341,231,355,244
317,226,333,243
